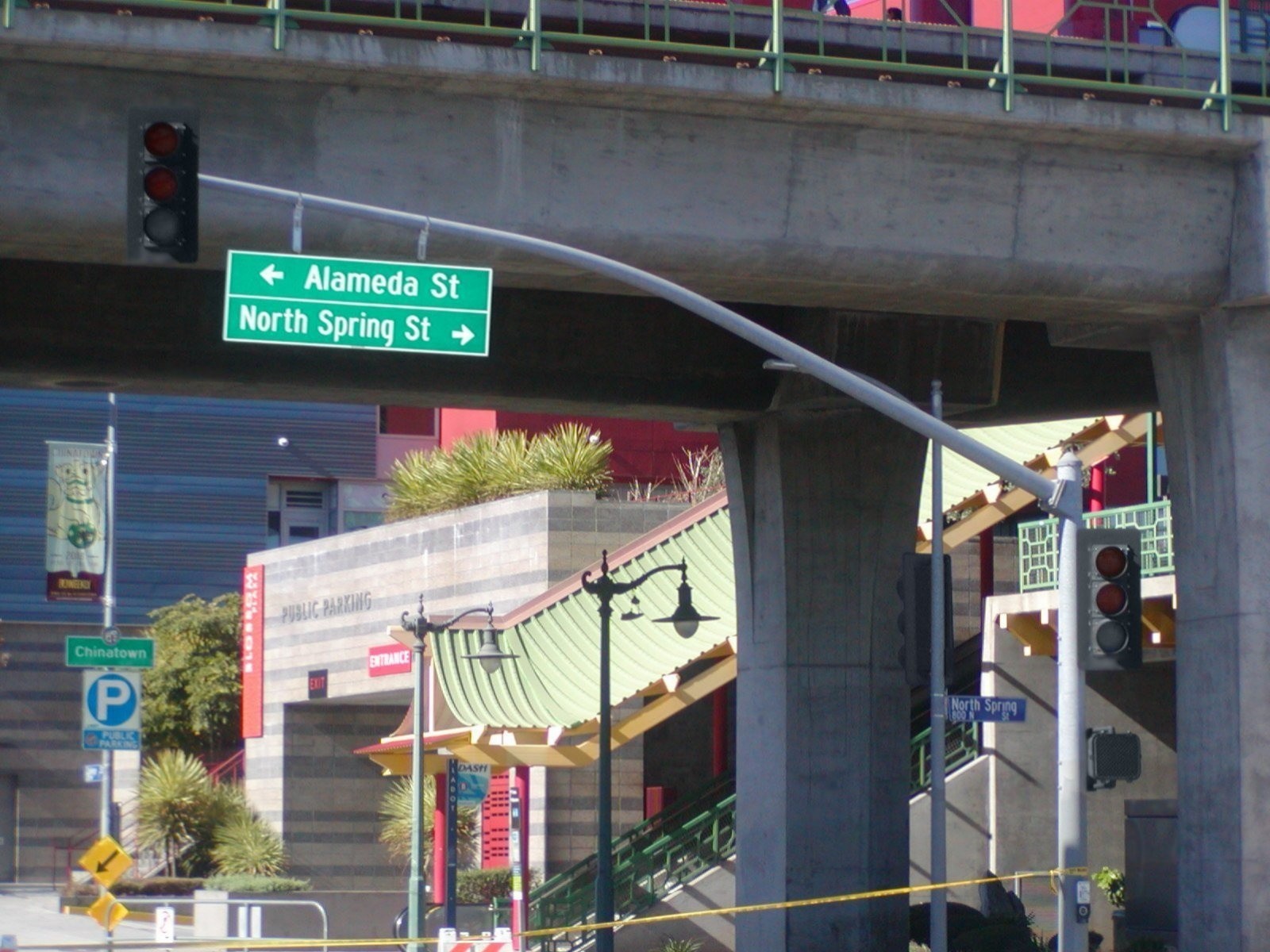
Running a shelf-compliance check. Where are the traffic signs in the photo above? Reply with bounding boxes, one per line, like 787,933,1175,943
222,251,499,355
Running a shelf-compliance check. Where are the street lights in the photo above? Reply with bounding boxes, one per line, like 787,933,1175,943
583,545,722,952
398,592,521,942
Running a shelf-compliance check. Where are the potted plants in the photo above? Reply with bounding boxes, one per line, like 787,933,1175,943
1089,865,1125,952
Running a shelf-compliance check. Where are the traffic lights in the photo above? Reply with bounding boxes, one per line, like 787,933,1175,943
121,101,199,262
896,553,953,686
1078,526,1143,672
1085,730,1141,783
